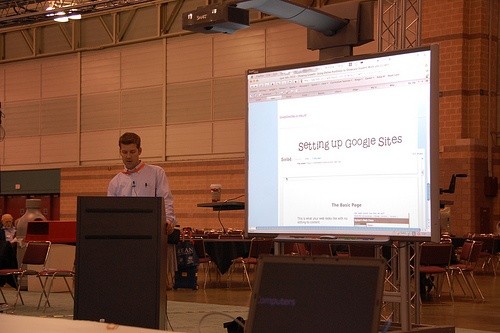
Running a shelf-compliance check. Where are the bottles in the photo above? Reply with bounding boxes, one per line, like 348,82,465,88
16,199,47,291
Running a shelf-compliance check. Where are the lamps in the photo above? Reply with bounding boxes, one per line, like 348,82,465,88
45,2,81,22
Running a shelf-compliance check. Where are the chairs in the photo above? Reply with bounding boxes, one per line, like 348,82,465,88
178,227,500,304
36,261,76,314
0,241,52,310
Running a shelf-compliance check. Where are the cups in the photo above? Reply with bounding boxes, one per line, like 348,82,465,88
210,184,222,201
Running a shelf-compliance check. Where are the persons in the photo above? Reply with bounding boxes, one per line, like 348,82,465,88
0,214,18,288
107,132,175,234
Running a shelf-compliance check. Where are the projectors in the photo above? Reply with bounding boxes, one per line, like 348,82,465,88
182,5,249,34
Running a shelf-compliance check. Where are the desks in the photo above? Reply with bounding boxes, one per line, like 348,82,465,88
467,237,500,255
382,237,466,303
203,239,274,288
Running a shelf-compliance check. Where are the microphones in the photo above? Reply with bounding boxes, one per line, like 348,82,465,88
133,181,135,184
